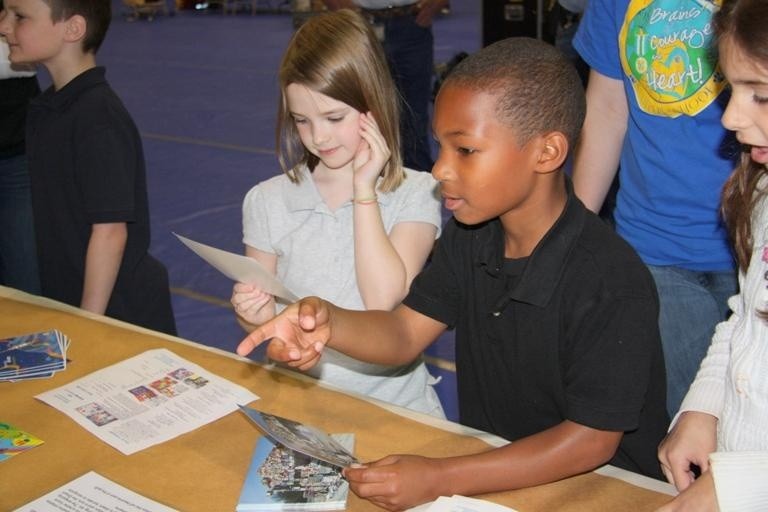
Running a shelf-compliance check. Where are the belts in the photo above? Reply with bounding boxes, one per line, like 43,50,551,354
360,4,420,19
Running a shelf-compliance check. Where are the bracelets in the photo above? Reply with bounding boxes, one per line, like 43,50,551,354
350,193,378,206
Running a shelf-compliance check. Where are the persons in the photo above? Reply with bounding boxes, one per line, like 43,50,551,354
0,34,41,297
237,38,670,511
657,0,768,512
229,8,446,421
1,0,177,335
570,0,739,421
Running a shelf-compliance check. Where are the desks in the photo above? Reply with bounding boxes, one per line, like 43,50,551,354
0,286,680,512
